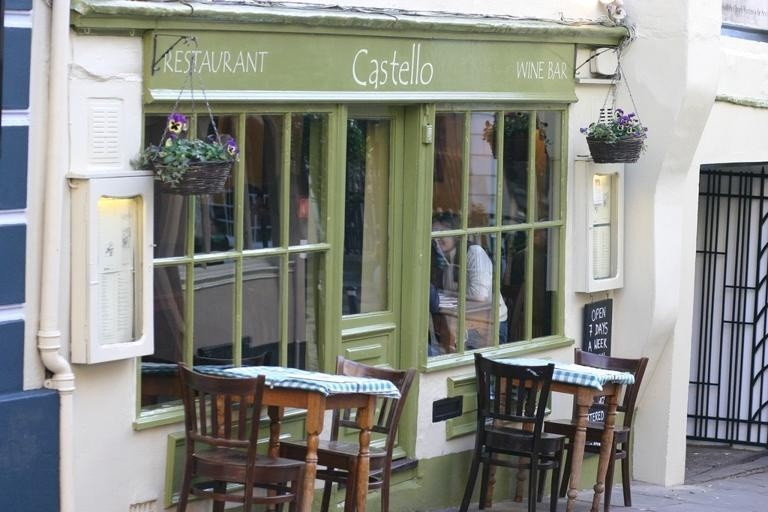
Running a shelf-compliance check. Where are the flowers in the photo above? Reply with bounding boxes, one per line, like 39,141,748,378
580,109,648,146
129,113,239,188
485,110,549,143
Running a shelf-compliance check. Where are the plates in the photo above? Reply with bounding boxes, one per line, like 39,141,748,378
439,293,458,308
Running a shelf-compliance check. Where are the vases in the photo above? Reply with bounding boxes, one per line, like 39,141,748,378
505,139,529,161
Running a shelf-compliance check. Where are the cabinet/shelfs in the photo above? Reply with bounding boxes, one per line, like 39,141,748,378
65,170,155,365
573,157,624,292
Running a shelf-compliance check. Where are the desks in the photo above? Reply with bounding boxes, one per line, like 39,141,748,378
217,365,402,512
483,358,636,512
434,289,501,354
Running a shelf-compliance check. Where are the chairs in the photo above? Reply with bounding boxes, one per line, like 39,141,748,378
279,355,418,512
179,362,306,512
458,353,568,512
536,348,649,512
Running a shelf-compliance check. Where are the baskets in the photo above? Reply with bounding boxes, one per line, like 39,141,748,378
486,130,528,161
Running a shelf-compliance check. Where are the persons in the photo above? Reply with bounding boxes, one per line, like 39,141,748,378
429,281,443,358
429,209,510,350
